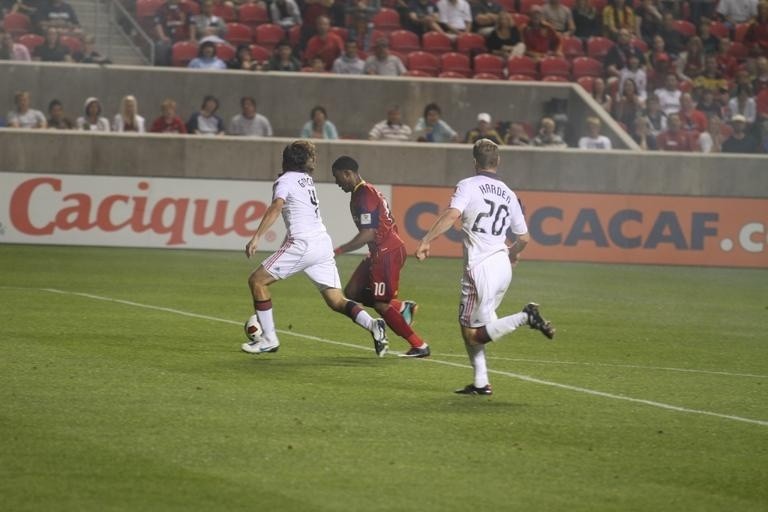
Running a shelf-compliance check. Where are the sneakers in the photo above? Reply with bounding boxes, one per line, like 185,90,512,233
401,301,418,326
370,317,389,357
521,302,555,339
398,343,430,358
455,383,492,395
240,337,280,353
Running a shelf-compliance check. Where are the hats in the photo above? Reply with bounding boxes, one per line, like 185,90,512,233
730,114,746,122
84,97,98,110
477,113,491,123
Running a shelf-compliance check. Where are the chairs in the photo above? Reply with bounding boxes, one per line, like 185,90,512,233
1,1,767,152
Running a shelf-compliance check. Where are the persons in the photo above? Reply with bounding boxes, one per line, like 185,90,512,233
0,1,602,78
328,154,431,359
577,116,612,150
366,103,413,142
75,97,111,132
461,111,503,145
228,96,273,137
602,0,768,153
46,99,73,131
299,105,338,141
529,117,568,149
185,94,226,137
239,140,390,360
502,123,531,146
112,95,146,133
2,90,48,130
412,138,556,397
414,102,461,143
148,96,189,135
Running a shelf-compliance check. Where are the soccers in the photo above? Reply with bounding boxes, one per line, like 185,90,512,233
244,314,263,342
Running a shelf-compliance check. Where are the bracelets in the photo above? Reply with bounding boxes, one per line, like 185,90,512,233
332,246,342,255
